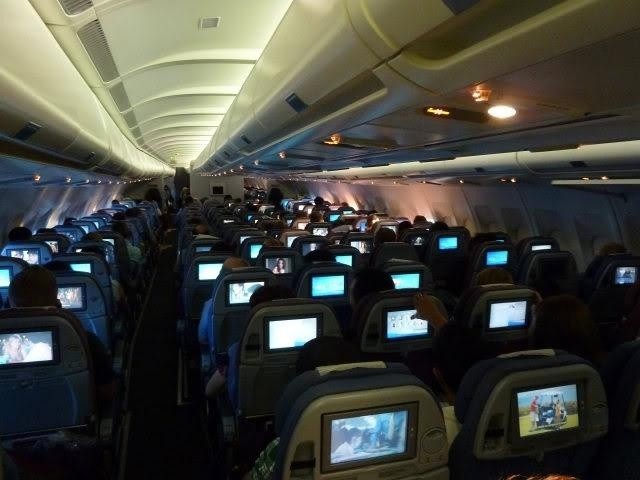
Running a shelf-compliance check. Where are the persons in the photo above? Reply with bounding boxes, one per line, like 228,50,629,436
110,221,141,262
9,265,118,411
311,196,330,214
530,395,539,429
203,283,290,395
245,335,358,480
556,400,562,428
398,220,412,239
232,282,263,299
272,259,286,274
332,428,361,458
410,292,491,446
261,239,283,248
413,215,425,225
305,212,323,231
373,227,396,246
346,267,394,334
81,246,123,304
191,225,210,237
196,256,250,346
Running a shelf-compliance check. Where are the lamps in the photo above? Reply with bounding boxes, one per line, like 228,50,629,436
486,102,520,120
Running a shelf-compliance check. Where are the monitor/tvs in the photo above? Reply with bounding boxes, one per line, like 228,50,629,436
263,313,323,353
66,261,93,273
334,239,341,245
335,253,354,268
194,244,212,253
0,327,58,368
312,227,329,236
238,235,257,245
0,266,13,289
6,248,40,265
43,239,60,253
484,248,510,267
349,239,373,254
343,211,353,215
410,235,425,248
328,214,340,222
360,223,365,232
530,243,551,252
196,262,224,281
321,402,419,473
382,225,396,233
309,273,347,298
225,279,268,307
615,267,637,285
286,234,299,247
56,283,87,312
300,241,323,256
263,255,294,276
248,243,262,262
510,382,583,445
382,307,432,342
437,234,460,254
487,298,530,332
389,271,422,289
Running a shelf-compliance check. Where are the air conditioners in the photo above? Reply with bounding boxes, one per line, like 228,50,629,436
147,148,169,164
123,110,138,129
109,81,132,112
135,137,145,146
131,126,142,138
76,18,121,84
200,16,221,29
140,144,149,150
57,0,94,17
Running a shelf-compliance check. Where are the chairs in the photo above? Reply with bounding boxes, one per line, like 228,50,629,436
368,241,420,271
291,261,353,312
449,348,609,480
0,256,30,307
81,232,144,320
393,216,409,224
0,240,54,267
181,198,258,241
231,230,267,253
52,270,126,379
326,234,345,245
515,235,559,264
79,216,107,230
327,211,343,223
181,238,224,278
31,233,71,256
379,258,433,291
468,241,516,274
119,197,160,228
397,227,426,260
270,361,449,480
198,265,277,378
449,225,471,242
577,252,640,331
53,252,126,338
338,206,355,215
374,212,388,219
91,212,113,222
65,220,98,234
608,336,640,431
290,217,312,231
279,229,310,247
239,236,267,266
54,225,87,243
0,306,124,480
291,235,330,257
354,290,449,385
305,221,333,237
325,244,361,267
493,230,512,245
515,250,578,299
204,296,344,472
259,195,332,219
424,230,462,268
372,220,399,244
179,251,233,331
452,283,546,356
339,232,375,256
255,246,305,288
338,214,359,225
412,220,433,231
97,223,139,247
62,240,122,283
352,216,369,231
102,208,118,218
